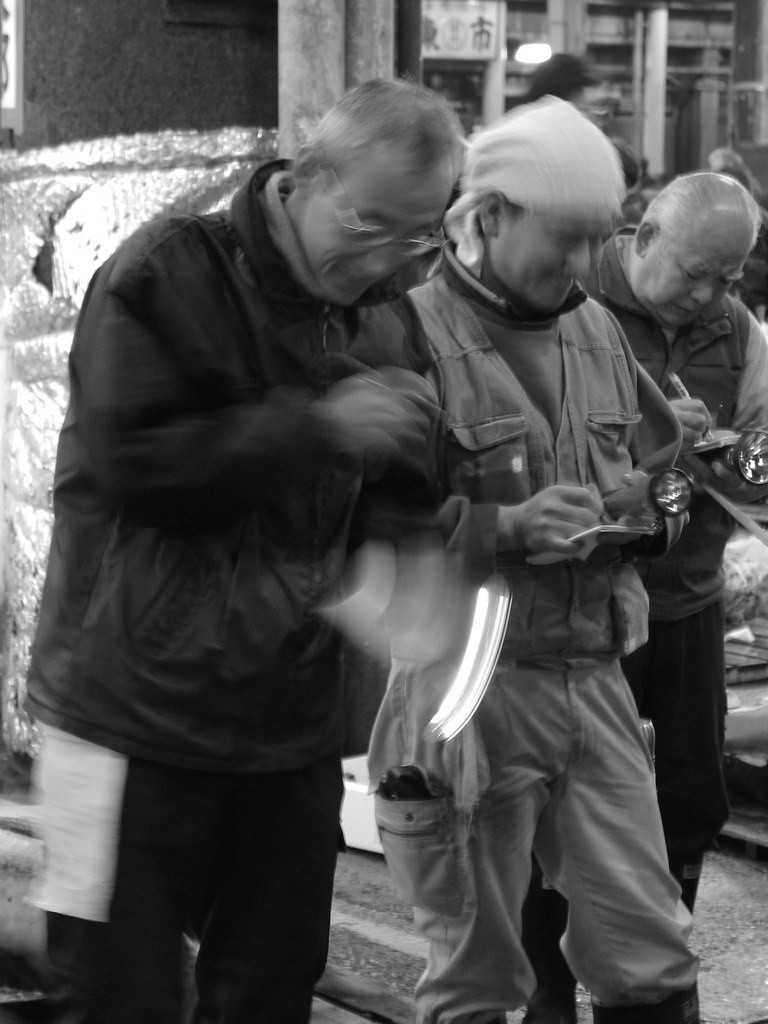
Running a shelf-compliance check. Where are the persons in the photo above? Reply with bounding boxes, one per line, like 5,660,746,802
23,79,462,1024
525,54,643,187
366,97,700,1024
526,172,768,1024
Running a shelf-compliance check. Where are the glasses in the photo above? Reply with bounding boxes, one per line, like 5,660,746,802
330,166,440,256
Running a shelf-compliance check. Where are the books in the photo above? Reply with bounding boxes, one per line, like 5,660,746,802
681,428,739,457
521,524,655,567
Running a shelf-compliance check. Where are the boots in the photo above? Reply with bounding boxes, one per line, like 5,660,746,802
520,872,577,1024
592,981,700,1024
667,852,704,915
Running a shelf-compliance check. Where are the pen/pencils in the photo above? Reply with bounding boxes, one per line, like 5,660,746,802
339,353,450,415
667,370,715,441
